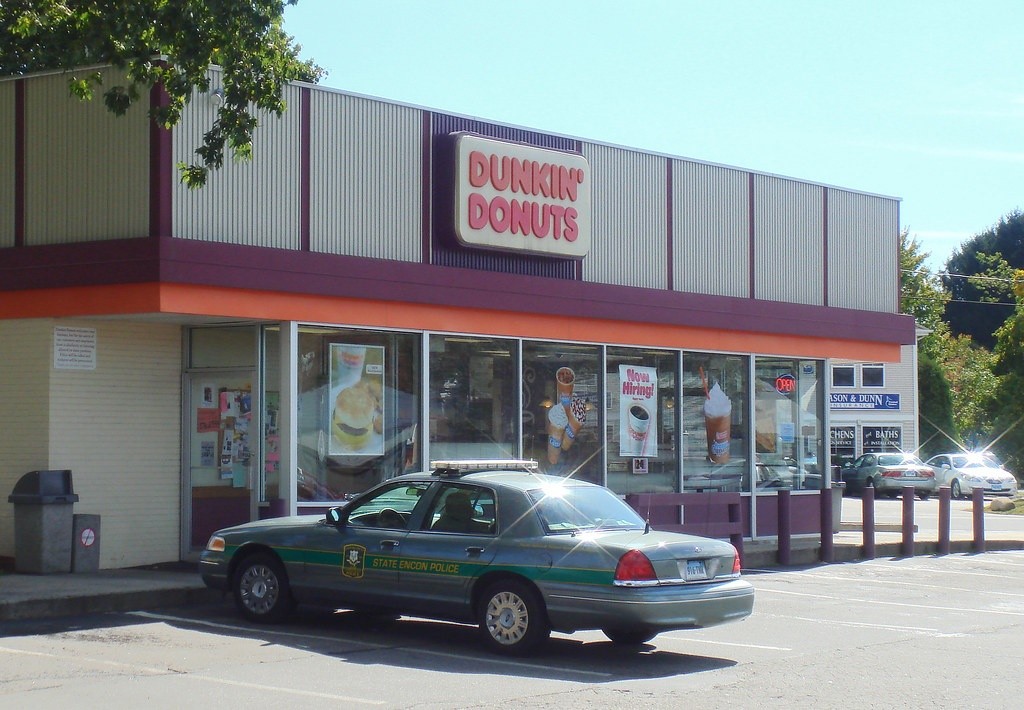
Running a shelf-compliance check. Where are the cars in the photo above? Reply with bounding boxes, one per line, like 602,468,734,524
924,454,1018,500
843,453,937,501
199,460,755,659
688,460,794,494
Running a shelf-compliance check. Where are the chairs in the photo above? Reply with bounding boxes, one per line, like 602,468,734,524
756,453,795,487
431,493,474,533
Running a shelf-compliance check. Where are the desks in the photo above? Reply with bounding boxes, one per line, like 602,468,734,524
672,473,744,493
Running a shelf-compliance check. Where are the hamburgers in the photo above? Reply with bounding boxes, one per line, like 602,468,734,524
332,387,376,448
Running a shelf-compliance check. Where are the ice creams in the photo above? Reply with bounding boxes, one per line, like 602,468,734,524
699,366,733,464
548,398,587,464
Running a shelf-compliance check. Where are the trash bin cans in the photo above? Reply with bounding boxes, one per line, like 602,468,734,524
830,465,848,534
73,513,101,572
7,469,80,575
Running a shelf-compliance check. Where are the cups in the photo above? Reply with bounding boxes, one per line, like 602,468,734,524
706,408,731,462
560,407,586,451
629,405,650,456
337,346,366,384
548,418,563,465
556,367,573,418
